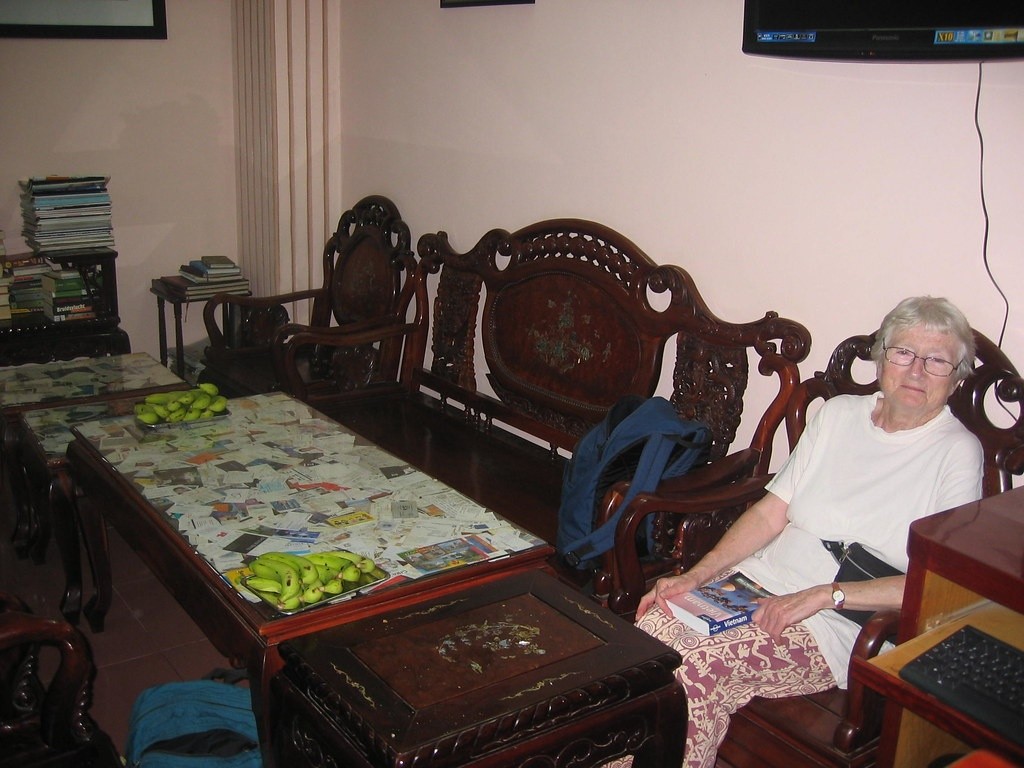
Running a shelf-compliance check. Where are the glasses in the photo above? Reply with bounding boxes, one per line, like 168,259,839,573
882,334,964,377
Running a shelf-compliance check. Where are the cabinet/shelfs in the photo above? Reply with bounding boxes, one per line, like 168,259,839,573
850,485,1024,767
0,248,131,368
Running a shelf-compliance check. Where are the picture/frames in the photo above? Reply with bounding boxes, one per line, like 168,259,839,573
0,0,168,40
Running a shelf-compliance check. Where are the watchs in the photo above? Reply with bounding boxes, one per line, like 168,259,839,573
831,582,844,610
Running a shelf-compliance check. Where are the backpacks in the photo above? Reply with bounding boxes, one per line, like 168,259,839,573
125,679,264,768
555,394,716,570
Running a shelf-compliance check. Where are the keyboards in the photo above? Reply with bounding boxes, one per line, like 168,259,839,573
899,625,1024,747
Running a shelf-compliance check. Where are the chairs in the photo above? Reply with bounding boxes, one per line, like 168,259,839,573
608,315,1024,768
1,574,121,768
198,196,423,442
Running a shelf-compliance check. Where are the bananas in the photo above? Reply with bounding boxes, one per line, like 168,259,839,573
134,382,228,424
245,551,376,610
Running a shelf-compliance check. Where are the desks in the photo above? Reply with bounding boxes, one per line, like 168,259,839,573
149,287,252,381
0,348,691,768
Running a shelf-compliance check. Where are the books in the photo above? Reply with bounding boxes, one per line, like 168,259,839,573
152,255,251,301
0,175,116,324
665,571,777,636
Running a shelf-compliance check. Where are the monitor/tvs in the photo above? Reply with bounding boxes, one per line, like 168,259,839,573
742,0,1024,65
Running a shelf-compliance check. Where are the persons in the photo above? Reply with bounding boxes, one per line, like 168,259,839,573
599,297,985,768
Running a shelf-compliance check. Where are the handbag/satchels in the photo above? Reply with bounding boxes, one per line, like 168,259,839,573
824,540,905,644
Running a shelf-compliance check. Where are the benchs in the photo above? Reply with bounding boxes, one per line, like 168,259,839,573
285,217,812,608
